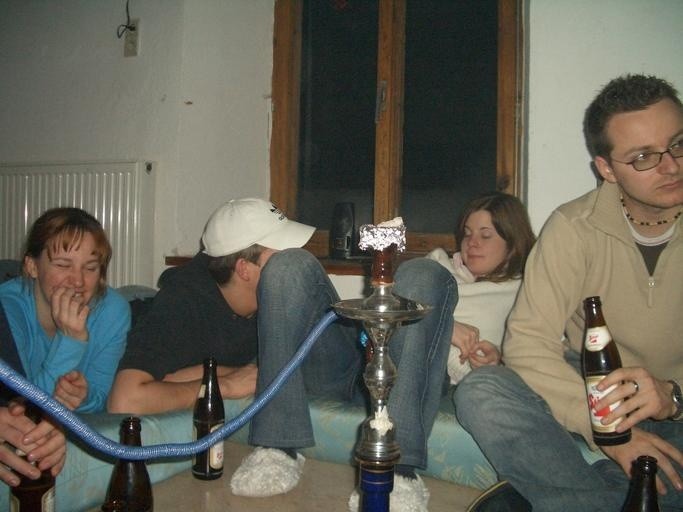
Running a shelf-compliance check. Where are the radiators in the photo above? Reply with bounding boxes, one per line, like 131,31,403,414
0,159,156,289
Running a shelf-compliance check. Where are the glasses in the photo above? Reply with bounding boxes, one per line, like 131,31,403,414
614,139,682,172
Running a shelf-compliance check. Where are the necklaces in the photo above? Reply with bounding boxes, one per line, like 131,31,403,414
620,190,683,227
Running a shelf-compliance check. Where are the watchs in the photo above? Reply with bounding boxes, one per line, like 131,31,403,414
666,379,683,421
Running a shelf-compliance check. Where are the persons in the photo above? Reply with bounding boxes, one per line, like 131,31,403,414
0,206,133,414
109,198,316,418
453,74,683,511
1,297,68,486
232,194,537,511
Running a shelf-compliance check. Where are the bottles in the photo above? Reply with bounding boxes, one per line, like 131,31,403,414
7,401,56,512
191,356,226,481
100,415,153,512
358,329,377,362
620,456,661,512
580,296,633,447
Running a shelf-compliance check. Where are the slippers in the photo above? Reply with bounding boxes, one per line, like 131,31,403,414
230,445,306,498
348,465,430,512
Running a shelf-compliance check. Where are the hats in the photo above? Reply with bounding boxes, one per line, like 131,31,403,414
201,197,318,260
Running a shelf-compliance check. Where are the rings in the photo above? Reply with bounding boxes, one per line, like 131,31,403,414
633,380,638,392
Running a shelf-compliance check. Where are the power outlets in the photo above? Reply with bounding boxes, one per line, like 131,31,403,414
124,19,138,57
